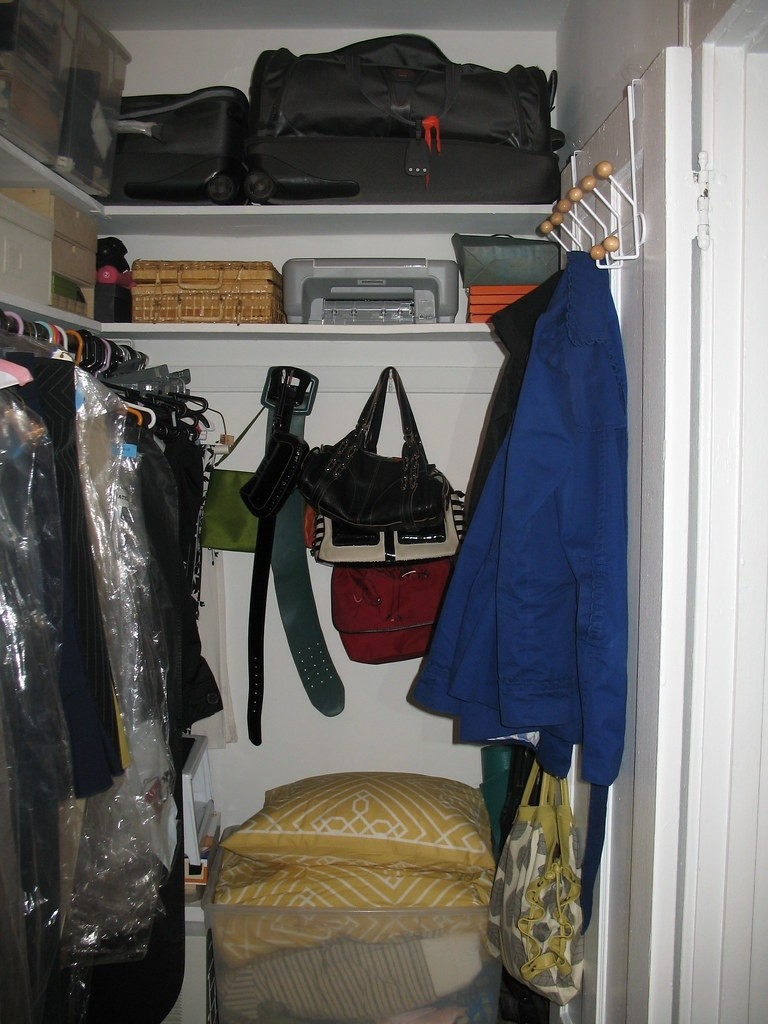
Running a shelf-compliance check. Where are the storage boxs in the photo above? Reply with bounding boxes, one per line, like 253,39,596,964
201,824,501,1024
0,0,134,200
0,195,57,306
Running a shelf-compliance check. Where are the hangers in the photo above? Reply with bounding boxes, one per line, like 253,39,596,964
1,306,213,446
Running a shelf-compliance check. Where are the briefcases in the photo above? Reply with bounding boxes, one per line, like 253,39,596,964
131,258,288,326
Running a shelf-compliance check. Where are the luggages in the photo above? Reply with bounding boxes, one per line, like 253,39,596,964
92,85,250,205
247,135,566,207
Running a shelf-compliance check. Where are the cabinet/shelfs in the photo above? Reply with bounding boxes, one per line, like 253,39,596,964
0,187,97,320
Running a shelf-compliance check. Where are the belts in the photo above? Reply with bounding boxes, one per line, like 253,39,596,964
260,366,345,718
246,370,302,747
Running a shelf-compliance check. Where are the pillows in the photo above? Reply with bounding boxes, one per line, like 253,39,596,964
214,770,501,871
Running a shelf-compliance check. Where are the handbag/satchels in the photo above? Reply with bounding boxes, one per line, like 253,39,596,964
246,29,569,154
450,233,561,288
297,366,445,533
484,758,585,1006
239,369,310,520
331,558,455,665
200,406,282,553
310,467,466,569
